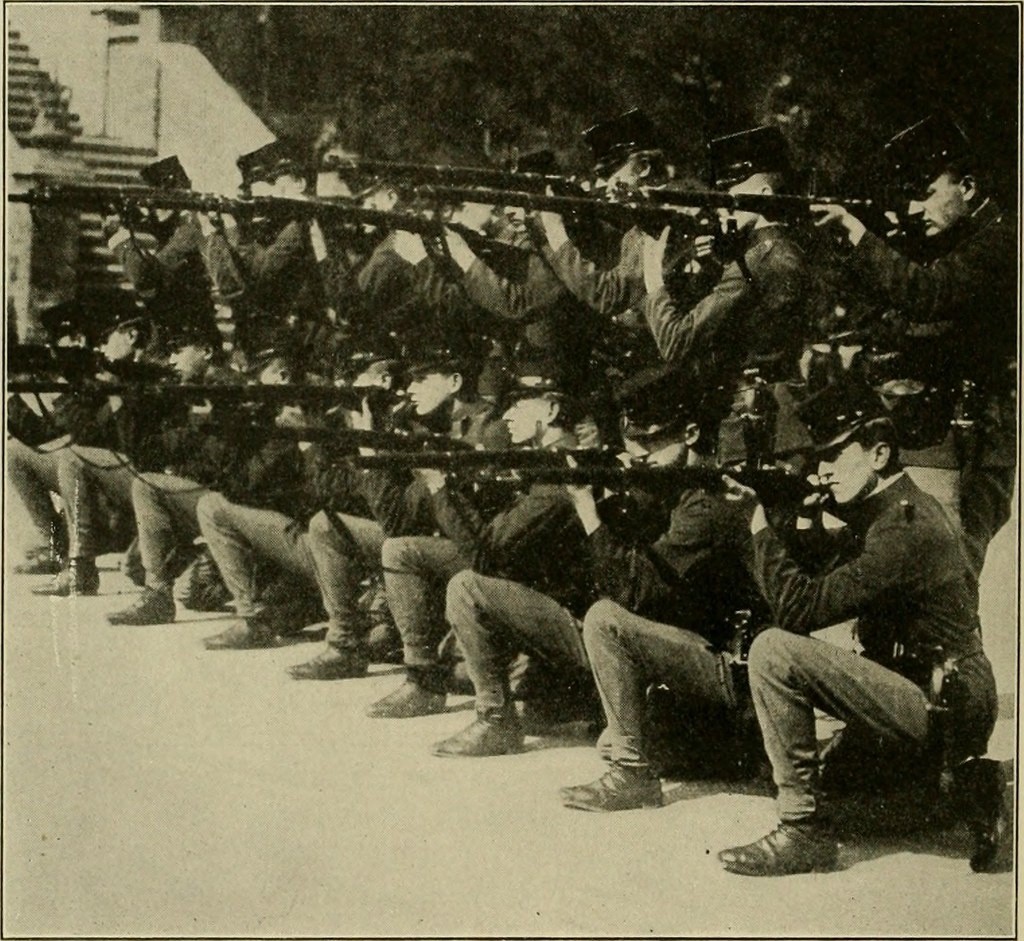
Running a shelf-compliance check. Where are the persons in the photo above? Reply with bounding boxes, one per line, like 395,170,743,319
717,380,1006,876
3,118,1016,813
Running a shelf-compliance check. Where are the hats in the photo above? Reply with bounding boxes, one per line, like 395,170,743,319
338,168,390,203
241,336,297,375
883,114,972,199
506,149,564,177
37,299,89,346
403,338,471,372
705,126,786,188
88,292,148,349
790,379,895,456
581,108,658,177
235,137,297,191
140,154,192,189
337,332,401,375
158,312,220,351
494,361,570,400
614,364,690,439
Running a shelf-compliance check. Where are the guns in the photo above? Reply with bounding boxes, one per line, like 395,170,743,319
137,198,233,216
415,185,701,242
646,187,922,238
2,379,132,395
232,195,491,256
9,343,88,369
190,419,411,447
65,382,349,411
6,192,132,212
513,468,820,516
322,154,599,194
349,448,629,494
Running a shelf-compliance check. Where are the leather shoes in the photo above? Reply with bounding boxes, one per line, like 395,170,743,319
19,541,1005,878
